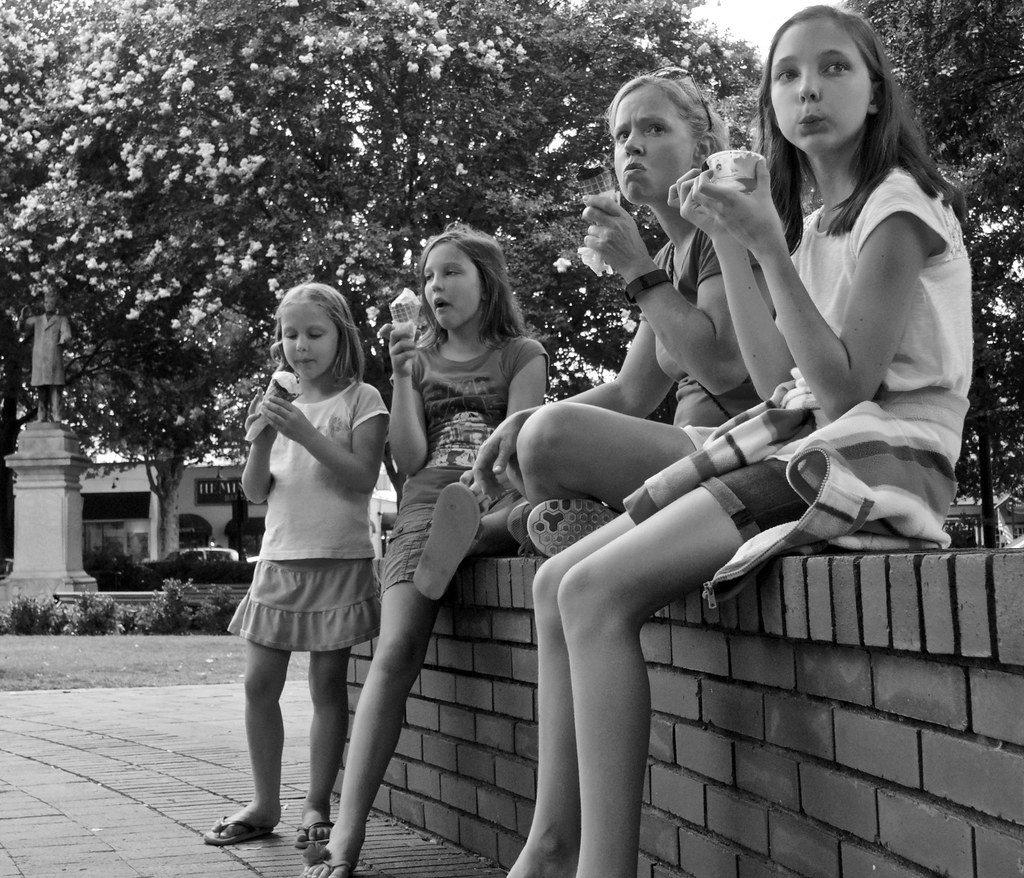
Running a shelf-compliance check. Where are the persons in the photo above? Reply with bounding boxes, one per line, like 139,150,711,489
472,80,775,557
16,290,73,421
205,284,389,849
508,5,974,878
302,222,551,878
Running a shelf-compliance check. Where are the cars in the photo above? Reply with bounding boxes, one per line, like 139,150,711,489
142,546,240,583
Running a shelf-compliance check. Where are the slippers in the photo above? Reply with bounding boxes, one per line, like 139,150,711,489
411,480,480,600
205,817,274,844
297,843,355,878
294,821,335,848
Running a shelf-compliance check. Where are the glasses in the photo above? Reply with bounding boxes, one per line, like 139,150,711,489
650,65,713,134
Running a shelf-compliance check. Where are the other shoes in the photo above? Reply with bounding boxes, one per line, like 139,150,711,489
528,497,620,558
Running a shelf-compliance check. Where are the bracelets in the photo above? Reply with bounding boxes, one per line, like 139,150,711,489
624,269,671,302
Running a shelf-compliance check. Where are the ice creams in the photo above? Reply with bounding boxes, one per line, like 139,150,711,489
263,370,303,404
389,288,422,326
706,149,767,191
576,165,616,195
